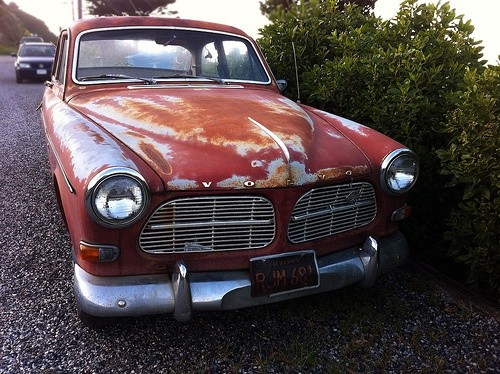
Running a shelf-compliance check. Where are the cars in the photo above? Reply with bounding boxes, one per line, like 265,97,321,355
17,36,44,46
39,15,421,329
11,41,59,82
